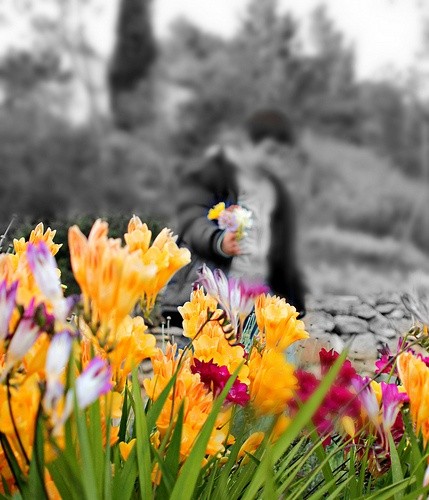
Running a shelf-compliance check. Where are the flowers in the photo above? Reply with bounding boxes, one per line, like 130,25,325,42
207,203,252,234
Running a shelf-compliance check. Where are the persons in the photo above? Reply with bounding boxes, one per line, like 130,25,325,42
162,106,308,328
